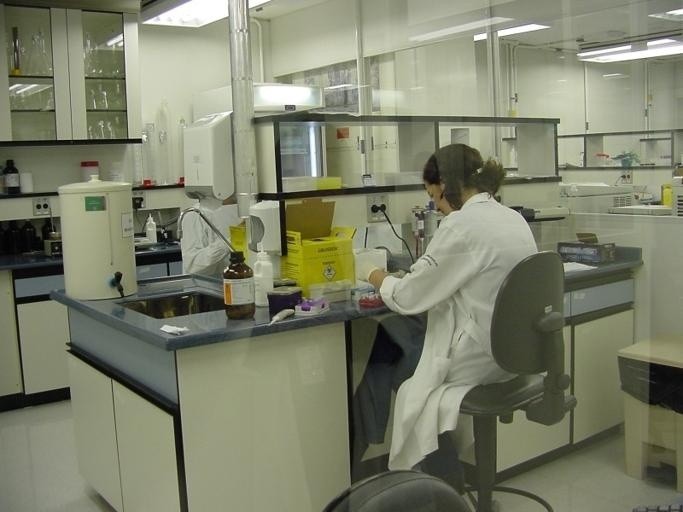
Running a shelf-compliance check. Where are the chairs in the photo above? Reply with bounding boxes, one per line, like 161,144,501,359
321,469,473,512
440,248,578,512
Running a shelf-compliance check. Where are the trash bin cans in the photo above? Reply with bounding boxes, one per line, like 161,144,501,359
616,336,682,494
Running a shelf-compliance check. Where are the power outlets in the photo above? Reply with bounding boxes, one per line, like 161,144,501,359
365,192,391,223
32,197,51,215
132,189,147,210
620,168,634,184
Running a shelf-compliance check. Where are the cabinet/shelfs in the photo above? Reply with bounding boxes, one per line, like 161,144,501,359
65,349,182,512
495,271,636,475
0,0,144,146
14,273,72,396
0,268,24,397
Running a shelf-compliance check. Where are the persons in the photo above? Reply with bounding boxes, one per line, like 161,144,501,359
177,190,243,280
352,142,539,498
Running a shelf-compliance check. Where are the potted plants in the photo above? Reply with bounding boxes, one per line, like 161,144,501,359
610,151,642,167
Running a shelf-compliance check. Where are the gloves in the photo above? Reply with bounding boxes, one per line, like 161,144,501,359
358,263,382,282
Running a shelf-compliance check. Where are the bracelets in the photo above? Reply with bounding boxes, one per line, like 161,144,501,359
366,267,381,283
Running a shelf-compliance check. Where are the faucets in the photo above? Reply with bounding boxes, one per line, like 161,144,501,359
111,271,126,297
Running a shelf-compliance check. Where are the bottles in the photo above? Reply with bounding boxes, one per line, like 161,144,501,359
508,145,518,167
144,213,157,245
3,159,19,193
0,217,60,259
253,243,275,308
4,22,126,140
0,166,8,195
79,160,100,182
137,115,187,185
223,251,255,320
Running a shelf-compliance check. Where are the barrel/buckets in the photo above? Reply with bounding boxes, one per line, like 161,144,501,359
56,175,140,301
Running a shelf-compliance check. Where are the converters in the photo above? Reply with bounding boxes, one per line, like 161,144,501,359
133,198,141,208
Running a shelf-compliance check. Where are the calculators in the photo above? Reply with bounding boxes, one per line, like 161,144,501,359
360,174,376,186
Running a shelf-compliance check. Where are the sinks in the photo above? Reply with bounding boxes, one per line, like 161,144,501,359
112,285,225,320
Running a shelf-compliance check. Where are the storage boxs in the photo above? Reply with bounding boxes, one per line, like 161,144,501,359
280,197,357,298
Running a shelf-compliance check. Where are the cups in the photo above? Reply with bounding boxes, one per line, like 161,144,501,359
18,170,33,192
108,161,123,182
